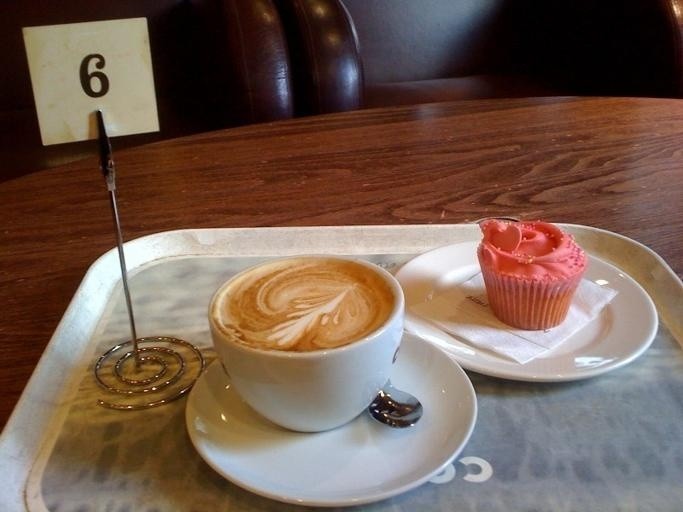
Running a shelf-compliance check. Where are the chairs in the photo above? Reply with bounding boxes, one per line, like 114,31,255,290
225,2,682,126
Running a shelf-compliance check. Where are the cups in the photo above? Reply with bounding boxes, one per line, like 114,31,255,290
206,251,408,434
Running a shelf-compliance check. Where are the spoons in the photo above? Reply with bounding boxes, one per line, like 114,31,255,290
366,373,425,429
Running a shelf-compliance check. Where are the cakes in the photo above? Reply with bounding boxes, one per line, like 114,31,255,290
476,216,586,330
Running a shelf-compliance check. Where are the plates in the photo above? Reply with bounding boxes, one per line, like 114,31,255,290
391,236,659,384
182,332,479,508
